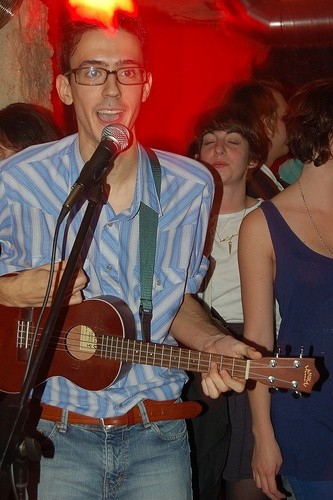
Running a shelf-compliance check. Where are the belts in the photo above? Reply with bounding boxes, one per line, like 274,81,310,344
27,399,203,430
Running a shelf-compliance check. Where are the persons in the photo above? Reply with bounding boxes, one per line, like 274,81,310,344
0,103,65,500
180,79,333,500
0,11,263,500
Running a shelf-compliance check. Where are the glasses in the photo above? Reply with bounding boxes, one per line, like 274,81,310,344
60,67,149,86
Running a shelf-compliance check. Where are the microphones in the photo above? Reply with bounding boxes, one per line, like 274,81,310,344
59,124,131,223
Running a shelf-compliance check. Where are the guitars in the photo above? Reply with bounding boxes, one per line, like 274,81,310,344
0,270,323,399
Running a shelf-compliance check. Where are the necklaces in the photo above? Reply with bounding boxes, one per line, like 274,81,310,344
297,178,333,257
212,197,248,254
